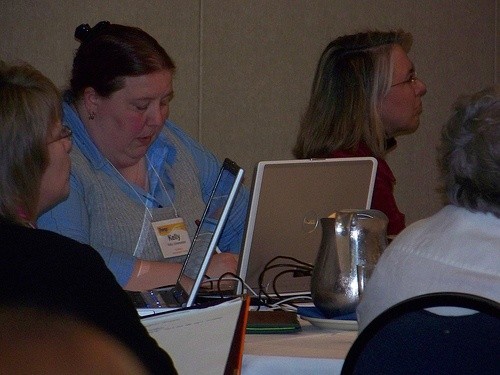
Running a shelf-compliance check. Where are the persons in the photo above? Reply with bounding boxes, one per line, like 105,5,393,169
0,57,179,375
291,27,427,243
356,85,499,331
36,19,258,294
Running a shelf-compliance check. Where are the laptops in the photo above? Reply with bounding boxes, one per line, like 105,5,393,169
113,157,245,318
198,157,377,299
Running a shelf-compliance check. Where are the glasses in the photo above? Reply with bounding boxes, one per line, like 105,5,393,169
391,71,417,87
42,125,75,154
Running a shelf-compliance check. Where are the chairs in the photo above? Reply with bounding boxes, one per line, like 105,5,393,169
340,292,500,375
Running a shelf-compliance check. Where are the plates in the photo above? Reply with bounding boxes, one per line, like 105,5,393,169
300,315,359,331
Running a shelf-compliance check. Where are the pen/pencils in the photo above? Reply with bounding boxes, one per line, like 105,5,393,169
195,219,221,253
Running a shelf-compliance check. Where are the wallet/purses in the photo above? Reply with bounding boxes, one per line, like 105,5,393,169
246,309,301,335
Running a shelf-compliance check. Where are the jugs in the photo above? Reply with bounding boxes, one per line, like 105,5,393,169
311,206,389,319
